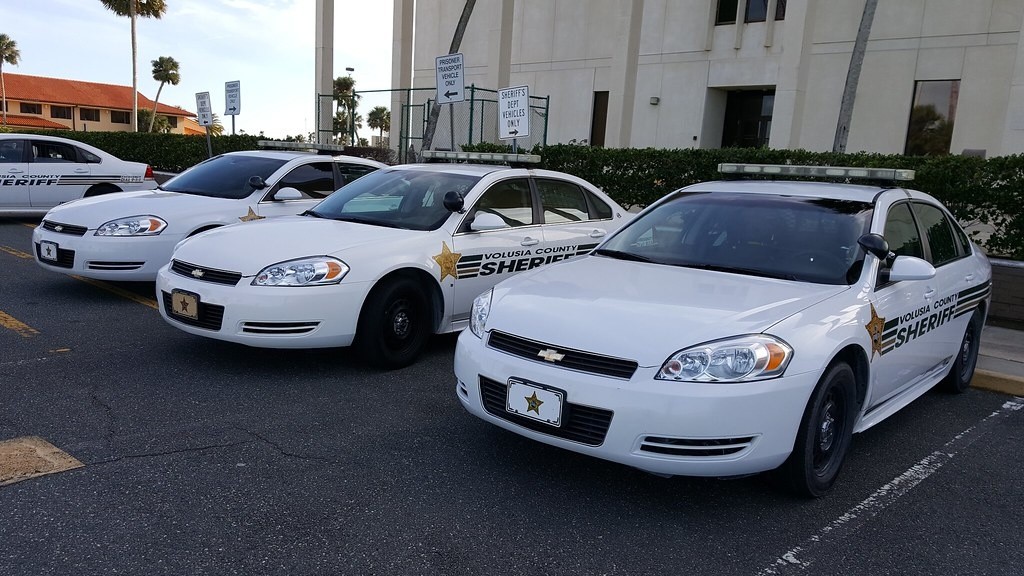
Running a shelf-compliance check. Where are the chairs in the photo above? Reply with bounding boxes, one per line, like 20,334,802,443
422,184,454,216
727,219,778,256
797,223,853,264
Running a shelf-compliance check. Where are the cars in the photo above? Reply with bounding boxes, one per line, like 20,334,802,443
455,163,989,500
31,142,433,283
0,132,159,218
156,162,651,366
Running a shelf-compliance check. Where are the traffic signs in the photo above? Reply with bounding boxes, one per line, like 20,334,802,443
435,53,465,104
497,85,531,140
196,90,213,127
223,80,241,117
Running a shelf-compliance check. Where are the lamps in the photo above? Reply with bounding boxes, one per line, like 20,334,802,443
650,97,659,105
